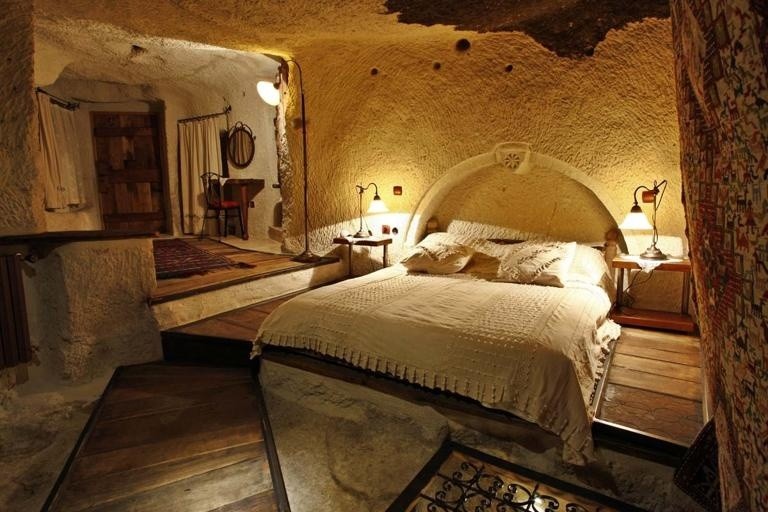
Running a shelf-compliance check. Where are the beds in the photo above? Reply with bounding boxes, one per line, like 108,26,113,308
251,242,622,468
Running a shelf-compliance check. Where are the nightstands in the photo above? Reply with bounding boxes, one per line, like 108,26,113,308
333,234,393,283
609,257,696,334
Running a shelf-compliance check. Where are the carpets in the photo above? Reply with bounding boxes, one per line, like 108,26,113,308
152,237,257,279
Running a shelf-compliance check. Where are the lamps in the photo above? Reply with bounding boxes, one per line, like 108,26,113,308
353,183,391,238
618,178,670,260
256,59,322,263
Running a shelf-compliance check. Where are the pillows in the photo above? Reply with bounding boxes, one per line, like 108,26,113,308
490,240,580,288
397,232,485,275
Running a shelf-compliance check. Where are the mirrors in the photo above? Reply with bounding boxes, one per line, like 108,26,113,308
226,121,257,170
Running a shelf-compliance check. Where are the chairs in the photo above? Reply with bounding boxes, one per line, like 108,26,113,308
198,172,245,241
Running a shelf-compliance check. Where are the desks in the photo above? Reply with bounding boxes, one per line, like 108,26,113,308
210,178,266,239
